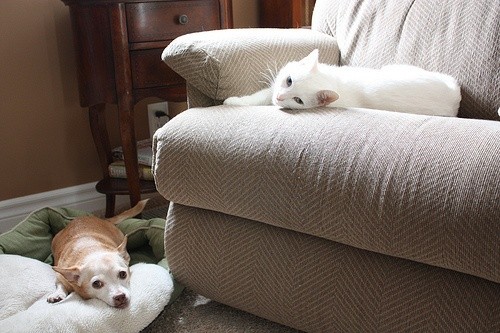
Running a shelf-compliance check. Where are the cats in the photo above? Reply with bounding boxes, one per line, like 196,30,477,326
223,49,462,117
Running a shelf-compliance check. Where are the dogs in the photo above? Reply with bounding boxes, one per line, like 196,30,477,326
47,199,149,309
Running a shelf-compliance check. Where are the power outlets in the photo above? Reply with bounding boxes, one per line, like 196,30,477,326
147,101,170,139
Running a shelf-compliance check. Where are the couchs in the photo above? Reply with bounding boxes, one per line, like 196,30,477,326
153,0,500,333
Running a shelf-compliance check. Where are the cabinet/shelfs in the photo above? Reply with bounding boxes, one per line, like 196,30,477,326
61,0,233,221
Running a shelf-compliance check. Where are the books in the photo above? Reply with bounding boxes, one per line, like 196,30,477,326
109,138,157,182
